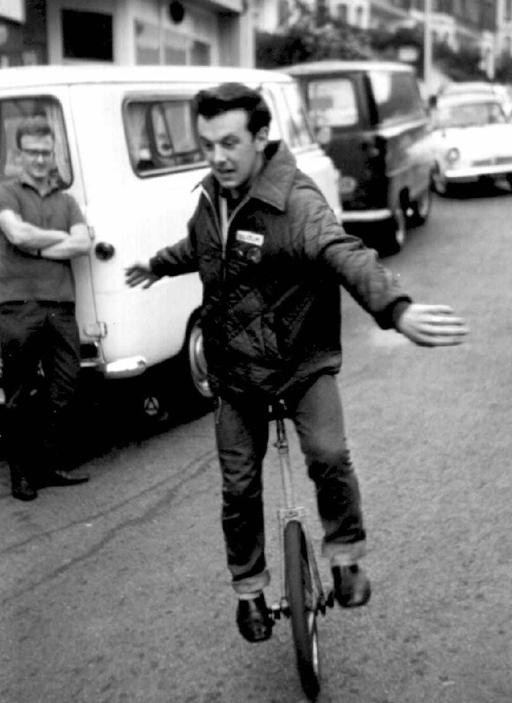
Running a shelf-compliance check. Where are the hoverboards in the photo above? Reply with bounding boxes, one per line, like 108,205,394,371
264,398,336,700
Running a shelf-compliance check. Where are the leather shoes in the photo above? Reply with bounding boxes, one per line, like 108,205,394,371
33,464,91,493
9,467,38,502
331,560,375,610
235,593,275,641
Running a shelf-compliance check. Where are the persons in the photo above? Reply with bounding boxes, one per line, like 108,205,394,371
125,81,469,641
0,116,92,501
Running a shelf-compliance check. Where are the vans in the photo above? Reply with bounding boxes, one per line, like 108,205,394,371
0,63,344,424
268,60,441,259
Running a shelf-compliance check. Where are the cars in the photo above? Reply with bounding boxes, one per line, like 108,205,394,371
426,79,511,197
